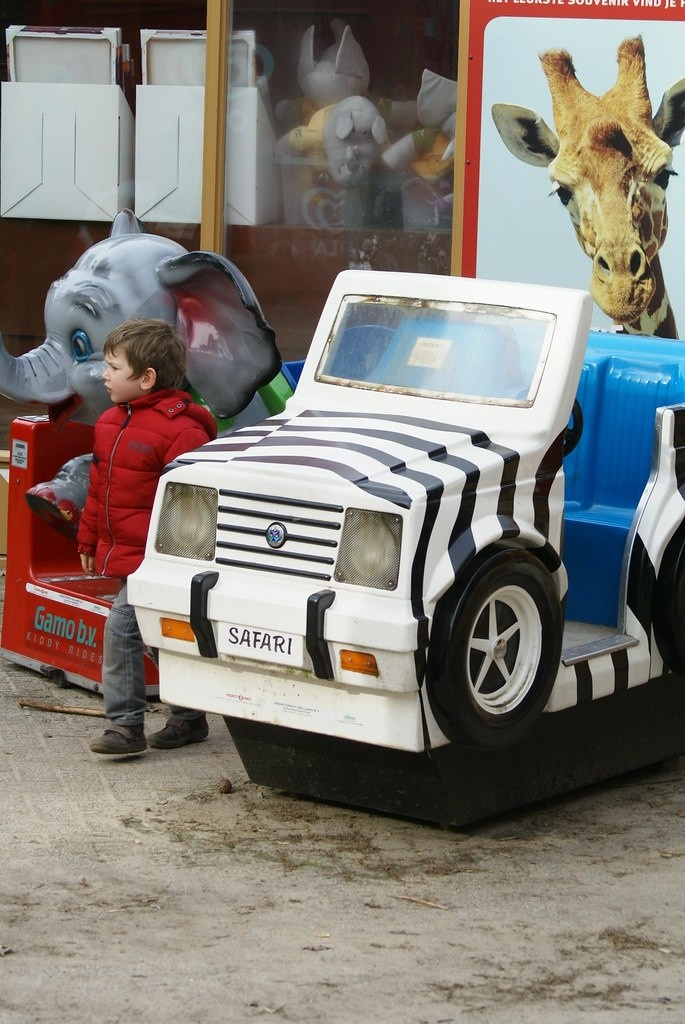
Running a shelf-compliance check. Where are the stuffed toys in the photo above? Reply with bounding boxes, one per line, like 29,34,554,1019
273,22,456,233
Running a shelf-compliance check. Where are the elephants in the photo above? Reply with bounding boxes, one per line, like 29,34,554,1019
1,207,283,540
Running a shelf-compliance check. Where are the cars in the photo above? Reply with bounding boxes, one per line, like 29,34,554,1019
123,265,684,831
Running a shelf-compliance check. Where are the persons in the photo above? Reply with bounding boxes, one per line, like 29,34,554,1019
76,319,218,754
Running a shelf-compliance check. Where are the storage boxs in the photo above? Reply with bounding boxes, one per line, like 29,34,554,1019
134,86,291,221
0,80,135,223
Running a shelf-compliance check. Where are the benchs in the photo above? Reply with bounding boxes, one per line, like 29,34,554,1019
369,320,684,629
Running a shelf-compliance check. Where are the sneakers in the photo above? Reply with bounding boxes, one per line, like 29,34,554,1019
146,709,210,749
91,725,148,755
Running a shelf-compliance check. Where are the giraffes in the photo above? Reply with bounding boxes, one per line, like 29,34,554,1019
492,35,685,341
